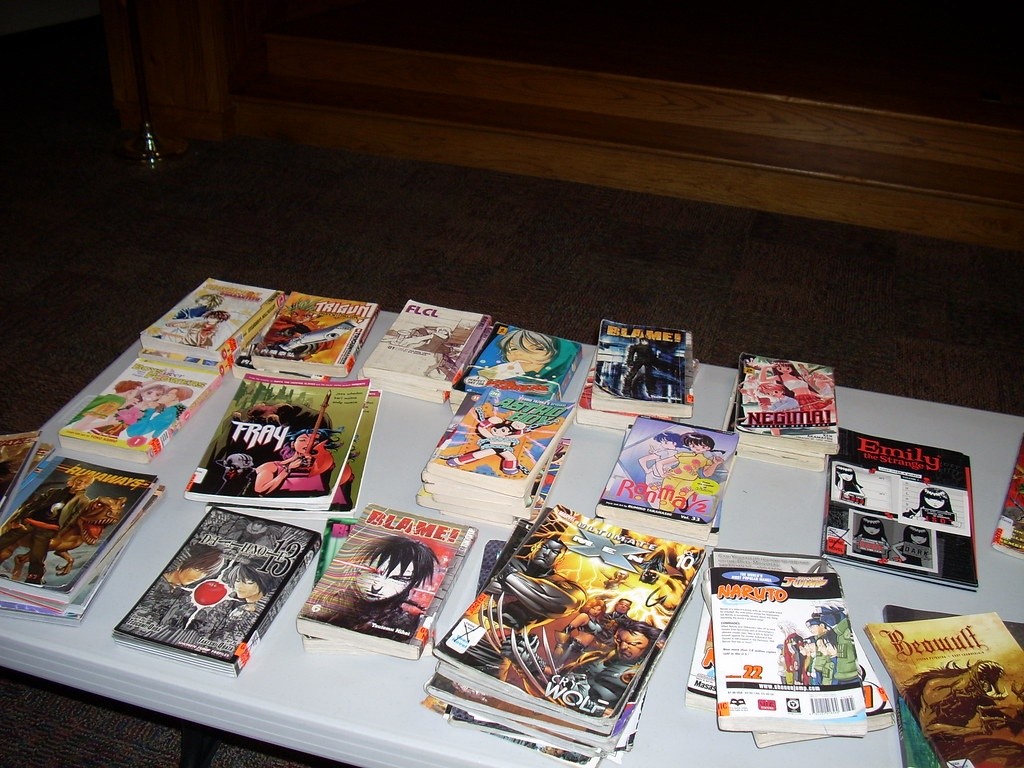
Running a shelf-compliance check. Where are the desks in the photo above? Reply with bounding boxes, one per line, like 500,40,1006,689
0,312,1024,768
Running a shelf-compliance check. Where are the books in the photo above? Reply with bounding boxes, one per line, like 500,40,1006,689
58,357,223,464
594,414,740,547
819,427,979,593
449,321,583,416
573,319,700,431
183,373,383,521
863,604,1024,768
684,547,896,750
722,351,841,472
420,502,707,768
111,507,322,678
0,430,168,628
415,387,577,530
990,433,1024,559
138,277,380,382
357,299,492,405
296,503,480,660
